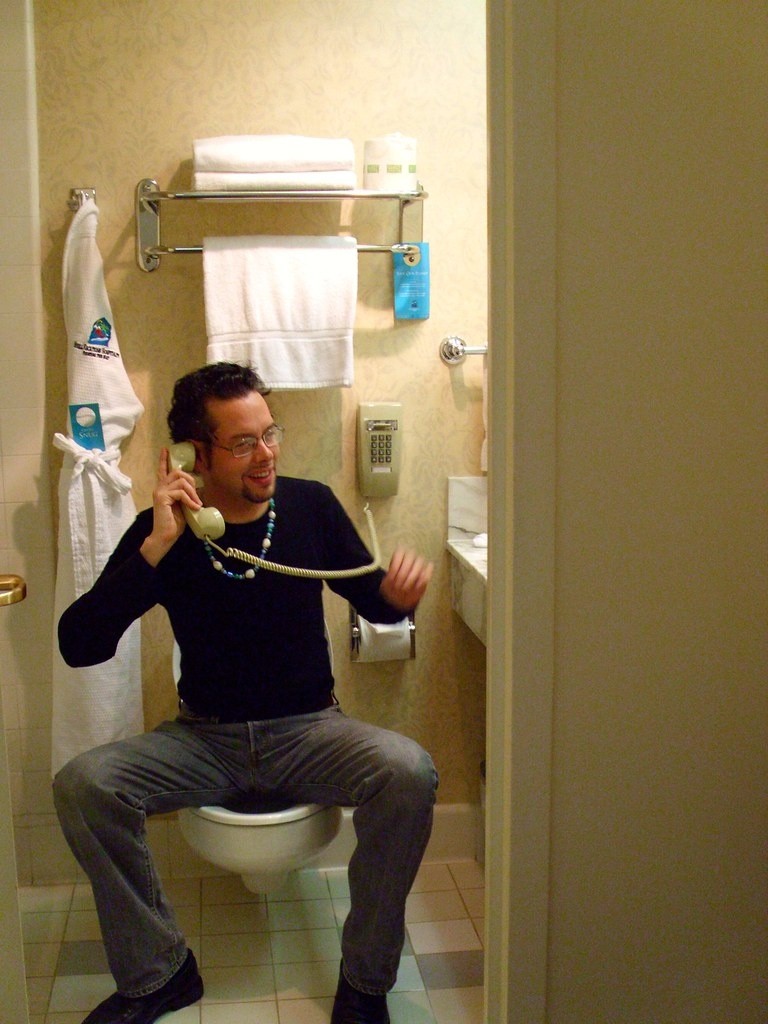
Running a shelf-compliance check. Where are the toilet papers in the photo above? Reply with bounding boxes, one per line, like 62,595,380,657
362,133,418,195
355,611,411,664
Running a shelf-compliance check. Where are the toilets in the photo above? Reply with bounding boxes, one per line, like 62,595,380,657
171,616,343,896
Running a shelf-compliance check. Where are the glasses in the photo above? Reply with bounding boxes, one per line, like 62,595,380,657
201,426,286,458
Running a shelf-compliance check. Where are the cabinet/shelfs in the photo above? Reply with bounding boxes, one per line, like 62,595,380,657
135,178,427,272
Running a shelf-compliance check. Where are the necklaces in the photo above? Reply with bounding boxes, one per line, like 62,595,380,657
204,498,276,579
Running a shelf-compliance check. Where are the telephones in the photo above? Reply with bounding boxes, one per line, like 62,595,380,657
167,441,226,539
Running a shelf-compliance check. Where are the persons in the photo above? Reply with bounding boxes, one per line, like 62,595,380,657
47,364,440,1024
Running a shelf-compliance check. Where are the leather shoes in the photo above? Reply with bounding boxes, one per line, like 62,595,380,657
80,949,204,1024
331,959,390,1024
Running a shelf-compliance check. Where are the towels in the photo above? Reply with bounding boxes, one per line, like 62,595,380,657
190,135,359,390
